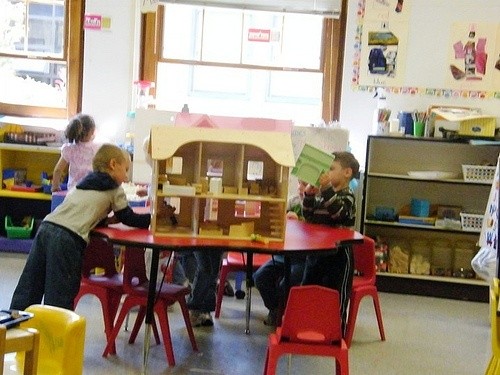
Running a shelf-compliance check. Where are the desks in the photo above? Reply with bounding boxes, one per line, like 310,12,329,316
93,219,364,375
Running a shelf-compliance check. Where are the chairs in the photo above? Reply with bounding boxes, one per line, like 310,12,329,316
63,229,385,375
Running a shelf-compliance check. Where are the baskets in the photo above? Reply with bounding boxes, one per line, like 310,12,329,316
4,214,34,239
459,210,485,231
461,164,496,182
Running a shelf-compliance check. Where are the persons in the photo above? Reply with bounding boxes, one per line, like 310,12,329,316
179,249,221,327
254,152,359,339
51,113,96,194
6,144,175,326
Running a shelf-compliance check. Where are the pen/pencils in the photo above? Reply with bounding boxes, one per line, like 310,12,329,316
411,108,436,123
377,109,391,123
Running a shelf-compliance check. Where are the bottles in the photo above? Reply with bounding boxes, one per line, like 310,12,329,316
387,234,482,280
397,113,414,136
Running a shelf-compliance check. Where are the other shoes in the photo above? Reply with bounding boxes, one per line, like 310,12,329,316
217,281,235,297
201,311,214,327
189,310,202,327
264,305,278,325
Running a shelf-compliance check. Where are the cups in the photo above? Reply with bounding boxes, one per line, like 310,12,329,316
377,122,390,136
424,121,435,137
389,118,399,133
414,121,424,137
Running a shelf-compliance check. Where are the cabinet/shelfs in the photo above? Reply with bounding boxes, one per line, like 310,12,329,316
0,139,75,253
362,132,500,303
148,128,294,242
